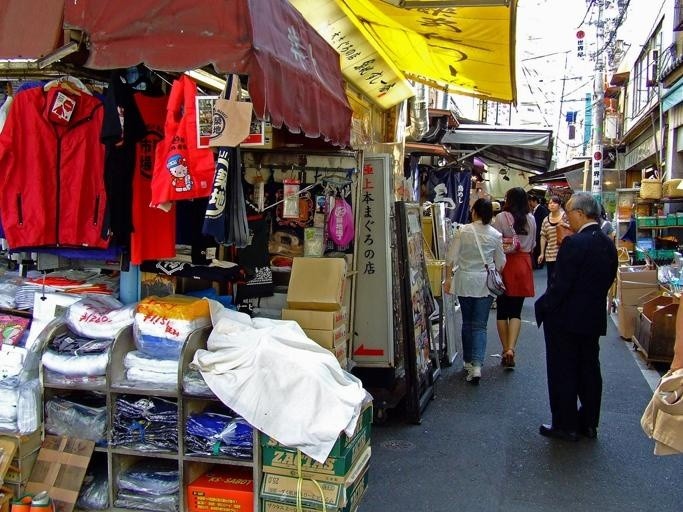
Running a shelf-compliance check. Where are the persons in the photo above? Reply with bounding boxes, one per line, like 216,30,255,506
490,189,614,292
442,199,507,383
669,288,683,375
495,187,536,365
534,189,618,442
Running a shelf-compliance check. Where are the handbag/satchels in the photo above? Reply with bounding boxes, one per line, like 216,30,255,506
487,271,506,295
503,237,521,255
556,225,574,245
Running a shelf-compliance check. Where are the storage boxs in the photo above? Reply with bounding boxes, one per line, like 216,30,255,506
425,259,442,297
281,256,348,372
0,425,42,501
259,391,373,512
420,216,433,259
616,265,659,341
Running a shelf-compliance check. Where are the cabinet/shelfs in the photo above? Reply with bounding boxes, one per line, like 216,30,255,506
632,289,679,369
38,322,262,512
635,192,683,265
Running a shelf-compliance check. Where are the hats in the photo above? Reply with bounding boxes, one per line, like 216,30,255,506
492,202,501,211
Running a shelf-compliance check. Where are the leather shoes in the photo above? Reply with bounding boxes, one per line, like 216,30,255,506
540,423,580,440
583,428,597,439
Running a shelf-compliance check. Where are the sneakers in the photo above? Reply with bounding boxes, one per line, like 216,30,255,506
502,348,515,367
462,361,482,383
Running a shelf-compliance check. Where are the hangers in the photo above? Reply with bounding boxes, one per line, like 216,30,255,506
44,64,81,97
64,65,82,92
323,171,354,183
